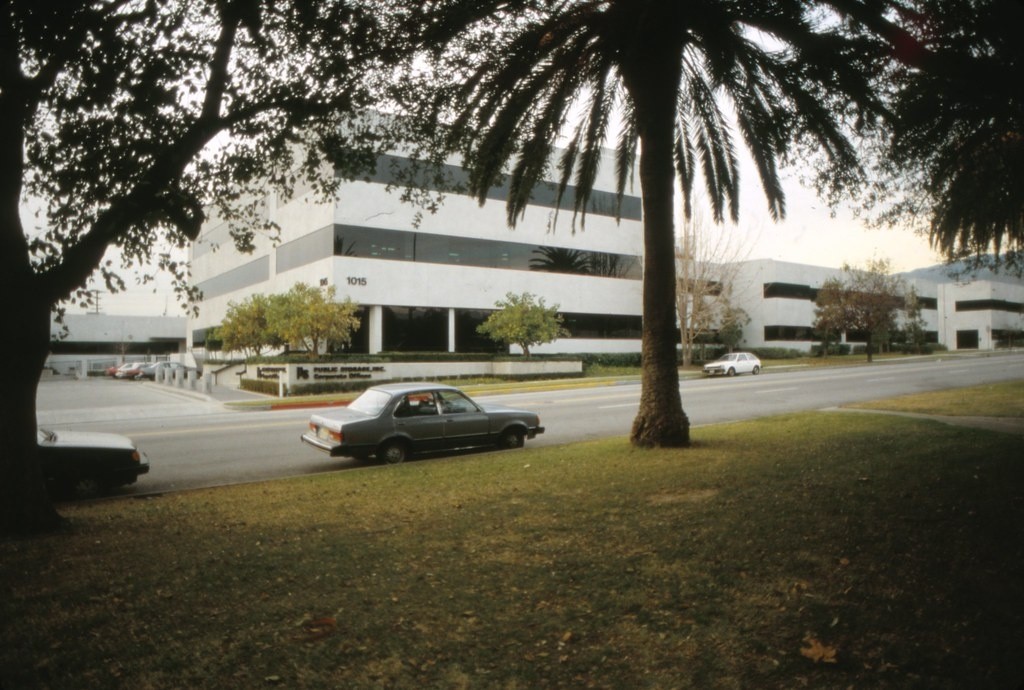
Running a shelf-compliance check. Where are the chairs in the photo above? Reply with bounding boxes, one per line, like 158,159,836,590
399,396,448,415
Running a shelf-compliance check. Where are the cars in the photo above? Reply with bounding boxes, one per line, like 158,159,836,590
701,351,763,377
115,362,154,381
36,427,150,499
139,361,202,381
300,381,545,464
105,363,125,378
